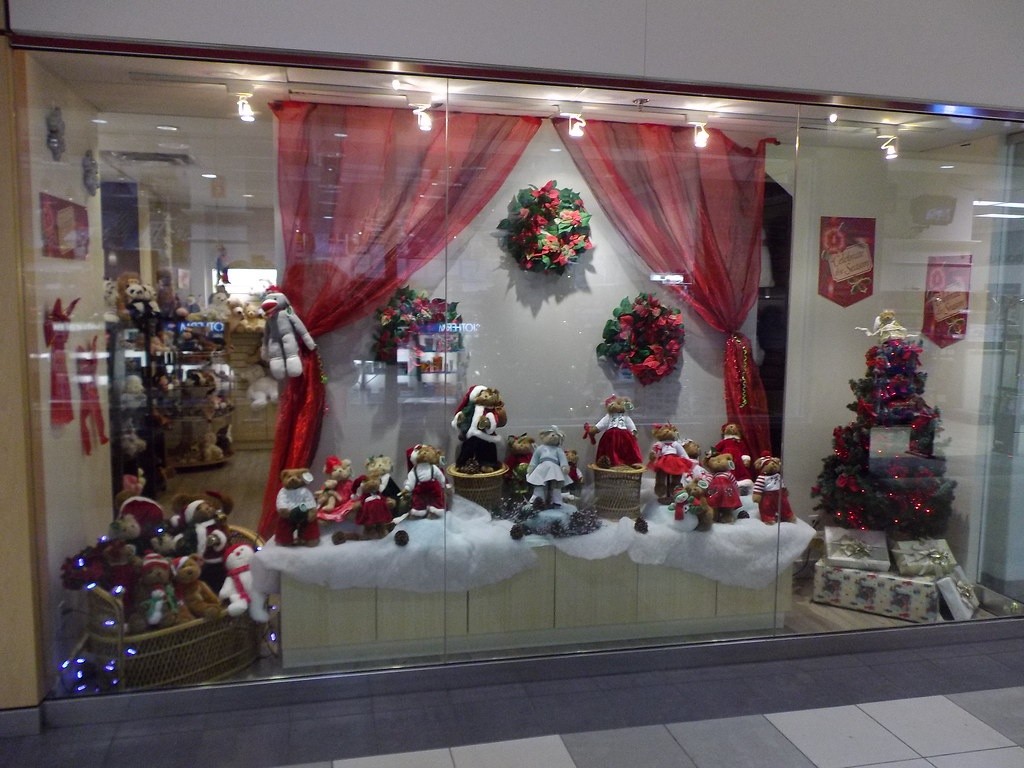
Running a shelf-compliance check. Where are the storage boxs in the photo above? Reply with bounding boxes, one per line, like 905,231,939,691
813,526,980,623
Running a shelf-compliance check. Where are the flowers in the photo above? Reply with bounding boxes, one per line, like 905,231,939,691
596,293,687,385
495,179,593,276
371,286,464,387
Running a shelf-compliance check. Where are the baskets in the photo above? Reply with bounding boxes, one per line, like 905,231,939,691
83,525,264,692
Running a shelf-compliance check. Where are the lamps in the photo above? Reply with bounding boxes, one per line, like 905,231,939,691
556,106,586,137
405,93,433,131
226,82,256,124
686,114,709,148
84,150,101,196
47,107,66,163
877,128,899,159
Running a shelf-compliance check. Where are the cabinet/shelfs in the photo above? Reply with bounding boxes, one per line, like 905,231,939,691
281,538,790,668
159,332,282,473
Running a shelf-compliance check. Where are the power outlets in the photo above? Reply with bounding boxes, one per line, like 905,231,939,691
808,516,821,531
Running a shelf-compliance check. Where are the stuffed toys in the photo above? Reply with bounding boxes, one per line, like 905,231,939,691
752,450,798,527
313,453,403,539
451,384,508,475
240,364,281,413
273,468,323,547
88,467,234,633
879,309,905,343
505,425,586,509
259,292,316,379
218,543,271,625
646,420,754,532
583,394,644,470
397,443,450,520
108,267,265,466
